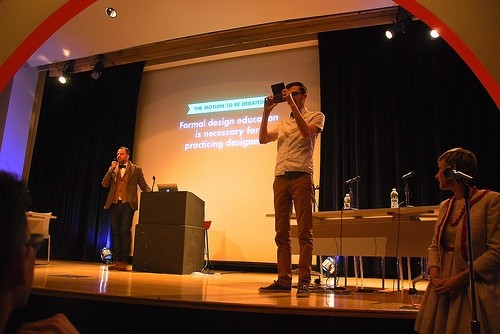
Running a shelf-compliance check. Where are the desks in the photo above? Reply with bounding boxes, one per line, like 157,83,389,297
265,206,444,295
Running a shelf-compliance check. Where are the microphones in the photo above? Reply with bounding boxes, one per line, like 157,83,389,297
402,171,415,180
443,167,475,182
346,176,360,184
151,176,155,191
314,185,321,190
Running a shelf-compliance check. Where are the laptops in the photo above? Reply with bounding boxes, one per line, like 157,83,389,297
157,184,178,191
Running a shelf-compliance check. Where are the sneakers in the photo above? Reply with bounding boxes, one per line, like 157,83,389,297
258,281,291,293
296,285,310,297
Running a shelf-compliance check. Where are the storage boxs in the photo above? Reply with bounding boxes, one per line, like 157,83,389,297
26,211,56,235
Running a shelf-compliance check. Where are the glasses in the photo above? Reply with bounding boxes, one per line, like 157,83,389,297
21,235,44,249
292,92,302,96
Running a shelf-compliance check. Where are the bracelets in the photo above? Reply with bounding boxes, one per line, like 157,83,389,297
430,276,439,280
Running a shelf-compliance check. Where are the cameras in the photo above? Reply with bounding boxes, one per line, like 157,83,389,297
272,82,287,102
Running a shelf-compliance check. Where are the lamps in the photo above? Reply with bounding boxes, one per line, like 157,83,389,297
90,55,105,79
384,5,413,39
57,60,75,84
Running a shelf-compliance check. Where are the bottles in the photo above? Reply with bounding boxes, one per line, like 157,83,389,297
390,188,398,209
344,194,350,210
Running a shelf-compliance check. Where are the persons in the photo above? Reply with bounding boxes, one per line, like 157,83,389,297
102,147,153,271
258,82,326,298
415,148,500,334
0,169,80,334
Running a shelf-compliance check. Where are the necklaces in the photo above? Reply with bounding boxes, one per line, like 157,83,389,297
447,185,477,226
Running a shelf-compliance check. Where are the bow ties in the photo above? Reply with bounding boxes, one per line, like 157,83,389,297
119,164,126,169
290,110,301,117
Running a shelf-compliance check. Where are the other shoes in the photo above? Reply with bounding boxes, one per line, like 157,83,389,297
109,262,126,270
